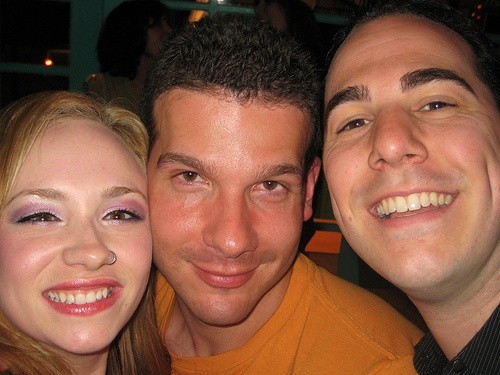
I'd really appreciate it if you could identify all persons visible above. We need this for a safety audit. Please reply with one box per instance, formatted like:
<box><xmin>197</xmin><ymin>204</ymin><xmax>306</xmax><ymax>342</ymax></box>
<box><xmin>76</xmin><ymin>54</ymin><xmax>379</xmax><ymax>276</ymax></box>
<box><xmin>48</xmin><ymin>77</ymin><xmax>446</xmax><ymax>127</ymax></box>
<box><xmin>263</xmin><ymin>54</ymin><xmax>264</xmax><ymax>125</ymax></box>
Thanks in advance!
<box><xmin>315</xmin><ymin>0</ymin><xmax>500</xmax><ymax>374</ymax></box>
<box><xmin>0</xmin><ymin>88</ymin><xmax>173</xmax><ymax>375</ymax></box>
<box><xmin>141</xmin><ymin>10</ymin><xmax>428</xmax><ymax>375</ymax></box>
